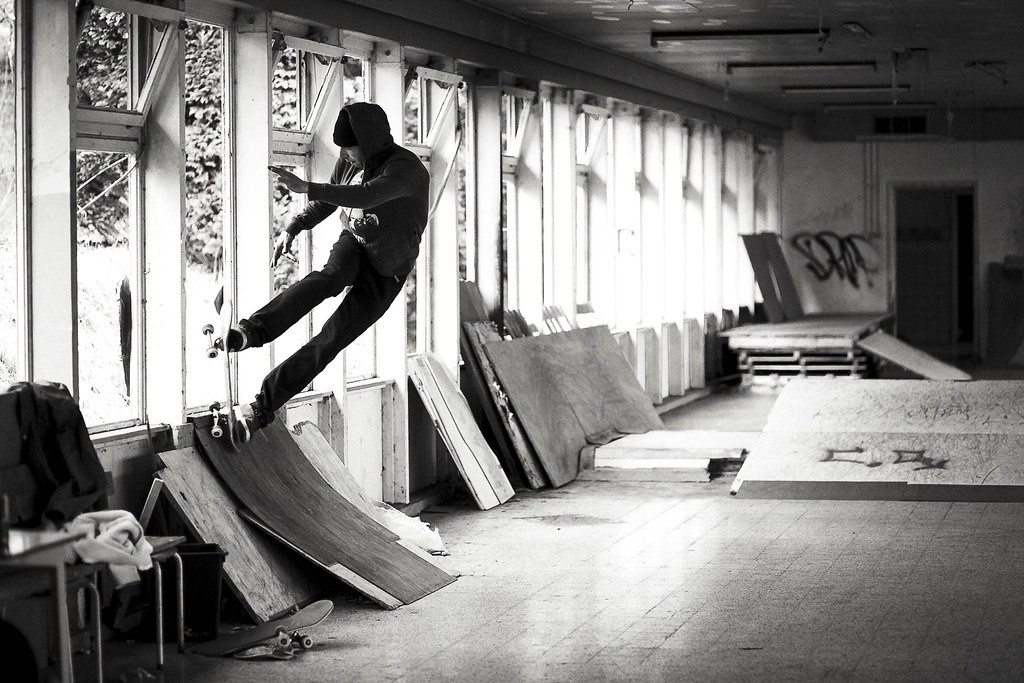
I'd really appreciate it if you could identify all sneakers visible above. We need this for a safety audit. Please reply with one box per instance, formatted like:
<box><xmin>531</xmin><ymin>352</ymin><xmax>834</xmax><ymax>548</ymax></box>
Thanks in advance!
<box><xmin>232</xmin><ymin>404</ymin><xmax>260</xmax><ymax>443</ymax></box>
<box><xmin>228</xmin><ymin>326</ymin><xmax>251</xmax><ymax>352</ymax></box>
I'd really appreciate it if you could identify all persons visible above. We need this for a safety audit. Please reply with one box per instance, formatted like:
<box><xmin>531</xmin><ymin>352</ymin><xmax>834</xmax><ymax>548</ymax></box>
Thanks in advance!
<box><xmin>229</xmin><ymin>102</ymin><xmax>431</xmax><ymax>444</ymax></box>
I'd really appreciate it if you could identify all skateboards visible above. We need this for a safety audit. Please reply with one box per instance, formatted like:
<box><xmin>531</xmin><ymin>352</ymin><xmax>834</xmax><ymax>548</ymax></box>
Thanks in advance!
<box><xmin>201</xmin><ymin>297</ymin><xmax>245</xmax><ymax>454</ymax></box>
<box><xmin>188</xmin><ymin>599</ymin><xmax>335</xmax><ymax>657</ymax></box>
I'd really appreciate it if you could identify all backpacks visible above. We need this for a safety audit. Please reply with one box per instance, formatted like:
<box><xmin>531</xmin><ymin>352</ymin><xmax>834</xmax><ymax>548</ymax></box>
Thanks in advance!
<box><xmin>0</xmin><ymin>379</ymin><xmax>109</xmax><ymax>530</ymax></box>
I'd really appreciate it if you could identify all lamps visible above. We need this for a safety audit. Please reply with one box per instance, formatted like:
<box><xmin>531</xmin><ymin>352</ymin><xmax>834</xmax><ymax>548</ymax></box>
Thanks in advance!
<box><xmin>649</xmin><ymin>29</ymin><xmax>834</xmax><ymax>47</ymax></box>
<box><xmin>824</xmin><ymin>103</ymin><xmax>936</xmax><ymax>112</ymax></box>
<box><xmin>781</xmin><ymin>87</ymin><xmax>909</xmax><ymax>97</ymax></box>
<box><xmin>726</xmin><ymin>62</ymin><xmax>879</xmax><ymax>74</ymax></box>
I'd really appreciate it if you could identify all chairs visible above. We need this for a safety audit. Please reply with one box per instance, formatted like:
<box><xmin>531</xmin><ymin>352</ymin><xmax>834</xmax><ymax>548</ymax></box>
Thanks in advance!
<box><xmin>0</xmin><ymin>379</ymin><xmax>185</xmax><ymax>683</ymax></box>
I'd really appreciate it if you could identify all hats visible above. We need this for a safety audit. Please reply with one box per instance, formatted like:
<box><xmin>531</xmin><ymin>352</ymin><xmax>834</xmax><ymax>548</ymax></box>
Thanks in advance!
<box><xmin>333</xmin><ymin>109</ymin><xmax>359</xmax><ymax>147</ymax></box>
